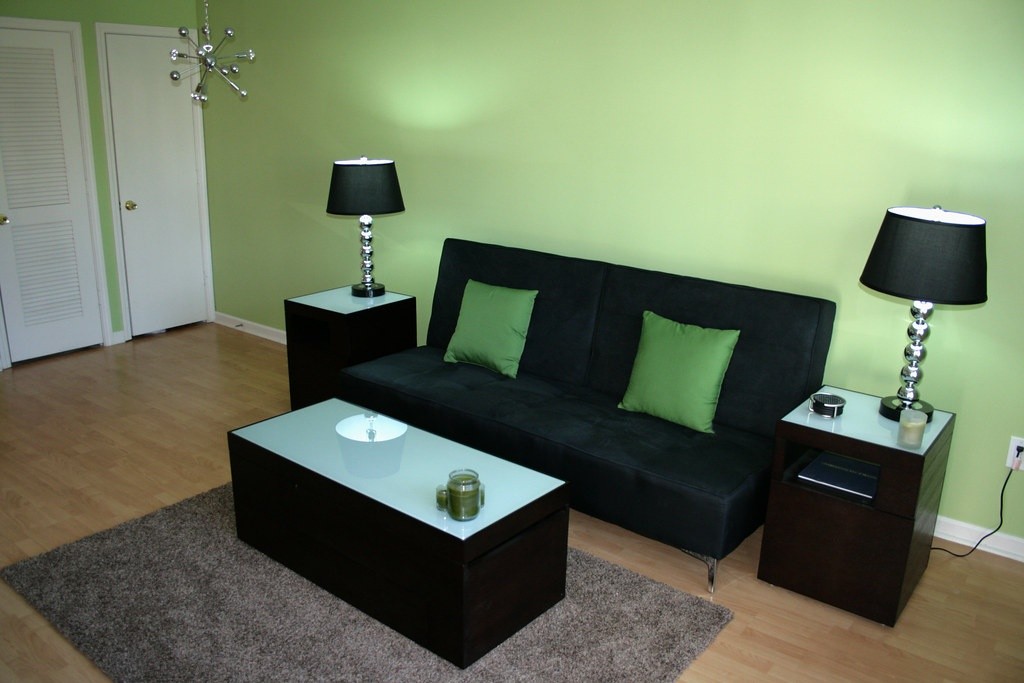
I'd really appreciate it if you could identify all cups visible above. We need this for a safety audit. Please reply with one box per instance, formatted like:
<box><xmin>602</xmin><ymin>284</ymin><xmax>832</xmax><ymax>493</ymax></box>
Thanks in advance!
<box><xmin>898</xmin><ymin>409</ymin><xmax>927</xmax><ymax>449</ymax></box>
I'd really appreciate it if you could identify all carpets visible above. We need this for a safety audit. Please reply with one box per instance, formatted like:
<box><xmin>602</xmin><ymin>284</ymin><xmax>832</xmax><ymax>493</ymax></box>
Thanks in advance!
<box><xmin>0</xmin><ymin>481</ymin><xmax>735</xmax><ymax>683</ymax></box>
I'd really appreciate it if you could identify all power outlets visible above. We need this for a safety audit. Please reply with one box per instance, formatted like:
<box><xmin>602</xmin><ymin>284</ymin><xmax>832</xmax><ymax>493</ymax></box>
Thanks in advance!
<box><xmin>1006</xmin><ymin>435</ymin><xmax>1024</xmax><ymax>471</ymax></box>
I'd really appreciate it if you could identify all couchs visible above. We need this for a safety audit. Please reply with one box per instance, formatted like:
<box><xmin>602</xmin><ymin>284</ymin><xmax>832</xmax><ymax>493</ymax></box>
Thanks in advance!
<box><xmin>333</xmin><ymin>238</ymin><xmax>837</xmax><ymax>595</ymax></box>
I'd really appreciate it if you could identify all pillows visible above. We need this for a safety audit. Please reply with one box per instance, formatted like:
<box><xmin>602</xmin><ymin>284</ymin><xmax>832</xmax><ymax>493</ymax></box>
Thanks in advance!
<box><xmin>618</xmin><ymin>311</ymin><xmax>741</xmax><ymax>433</ymax></box>
<box><xmin>442</xmin><ymin>278</ymin><xmax>539</xmax><ymax>381</ymax></box>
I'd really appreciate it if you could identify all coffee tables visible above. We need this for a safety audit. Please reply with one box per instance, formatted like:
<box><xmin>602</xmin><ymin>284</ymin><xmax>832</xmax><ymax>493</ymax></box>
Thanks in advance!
<box><xmin>226</xmin><ymin>398</ymin><xmax>573</xmax><ymax>673</ymax></box>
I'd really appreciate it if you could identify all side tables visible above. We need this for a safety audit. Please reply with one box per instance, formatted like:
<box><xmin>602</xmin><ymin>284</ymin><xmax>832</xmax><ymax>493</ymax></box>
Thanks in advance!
<box><xmin>283</xmin><ymin>285</ymin><xmax>418</xmax><ymax>411</ymax></box>
<box><xmin>756</xmin><ymin>385</ymin><xmax>957</xmax><ymax>629</ymax></box>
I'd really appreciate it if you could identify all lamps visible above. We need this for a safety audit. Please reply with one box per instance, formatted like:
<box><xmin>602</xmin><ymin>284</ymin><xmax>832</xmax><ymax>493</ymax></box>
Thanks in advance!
<box><xmin>169</xmin><ymin>0</ymin><xmax>257</xmax><ymax>102</ymax></box>
<box><xmin>335</xmin><ymin>410</ymin><xmax>408</xmax><ymax>480</ymax></box>
<box><xmin>325</xmin><ymin>156</ymin><xmax>406</xmax><ymax>298</ymax></box>
<box><xmin>859</xmin><ymin>204</ymin><xmax>989</xmax><ymax>423</ymax></box>
